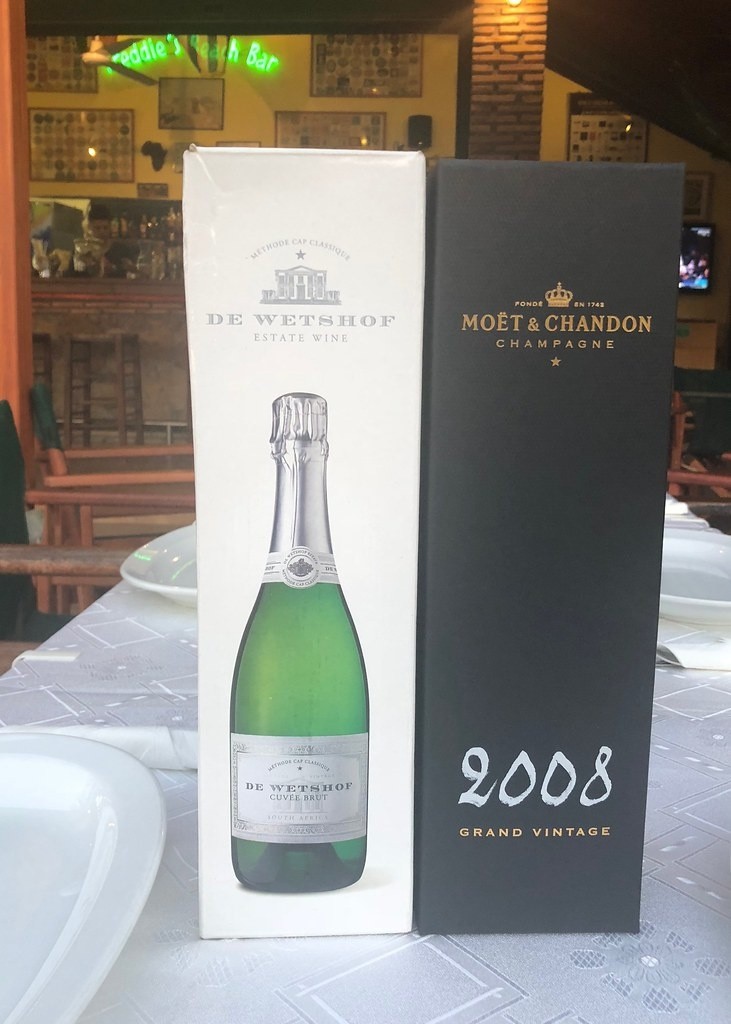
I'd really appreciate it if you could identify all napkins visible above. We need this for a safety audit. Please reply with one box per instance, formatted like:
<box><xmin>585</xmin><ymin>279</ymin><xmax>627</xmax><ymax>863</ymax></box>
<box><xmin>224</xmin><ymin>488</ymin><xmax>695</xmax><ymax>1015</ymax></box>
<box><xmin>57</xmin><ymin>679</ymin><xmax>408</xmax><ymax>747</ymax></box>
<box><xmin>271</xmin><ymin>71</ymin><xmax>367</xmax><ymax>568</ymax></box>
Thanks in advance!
<box><xmin>0</xmin><ymin>725</ymin><xmax>199</xmax><ymax>771</ymax></box>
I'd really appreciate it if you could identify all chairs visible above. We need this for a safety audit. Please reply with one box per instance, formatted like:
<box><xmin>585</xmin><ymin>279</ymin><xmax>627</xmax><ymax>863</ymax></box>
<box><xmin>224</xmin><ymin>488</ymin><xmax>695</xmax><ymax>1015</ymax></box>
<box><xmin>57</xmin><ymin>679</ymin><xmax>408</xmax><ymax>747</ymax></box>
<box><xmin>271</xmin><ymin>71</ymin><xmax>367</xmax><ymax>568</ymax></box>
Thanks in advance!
<box><xmin>666</xmin><ymin>388</ymin><xmax>731</xmax><ymax>534</ymax></box>
<box><xmin>0</xmin><ymin>383</ymin><xmax>197</xmax><ymax>676</ymax></box>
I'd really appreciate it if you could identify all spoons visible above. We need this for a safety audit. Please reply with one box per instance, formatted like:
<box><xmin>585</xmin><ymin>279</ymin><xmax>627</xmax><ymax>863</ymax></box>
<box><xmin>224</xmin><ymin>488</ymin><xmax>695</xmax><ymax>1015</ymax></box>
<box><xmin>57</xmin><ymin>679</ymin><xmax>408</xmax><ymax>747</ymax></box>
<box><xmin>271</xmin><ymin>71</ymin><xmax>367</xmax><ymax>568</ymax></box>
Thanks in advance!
<box><xmin>656</xmin><ymin>643</ymin><xmax>679</xmax><ymax>665</ymax></box>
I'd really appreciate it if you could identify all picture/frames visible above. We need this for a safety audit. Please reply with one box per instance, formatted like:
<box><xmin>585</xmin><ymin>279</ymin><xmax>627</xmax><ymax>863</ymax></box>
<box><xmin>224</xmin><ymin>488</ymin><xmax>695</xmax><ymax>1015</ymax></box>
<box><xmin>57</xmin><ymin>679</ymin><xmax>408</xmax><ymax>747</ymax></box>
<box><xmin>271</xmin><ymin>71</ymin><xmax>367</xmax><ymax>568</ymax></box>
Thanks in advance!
<box><xmin>681</xmin><ymin>171</ymin><xmax>713</xmax><ymax>224</ymax></box>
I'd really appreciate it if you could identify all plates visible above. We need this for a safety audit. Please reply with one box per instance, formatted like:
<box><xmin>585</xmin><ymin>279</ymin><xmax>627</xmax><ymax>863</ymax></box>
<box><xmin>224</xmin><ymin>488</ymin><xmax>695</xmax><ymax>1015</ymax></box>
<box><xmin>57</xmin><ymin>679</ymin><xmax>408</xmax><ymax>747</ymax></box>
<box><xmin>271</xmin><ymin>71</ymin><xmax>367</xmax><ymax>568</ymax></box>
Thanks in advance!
<box><xmin>120</xmin><ymin>523</ymin><xmax>196</xmax><ymax>606</ymax></box>
<box><xmin>0</xmin><ymin>731</ymin><xmax>167</xmax><ymax>1024</ymax></box>
<box><xmin>657</xmin><ymin>528</ymin><xmax>731</xmax><ymax>630</ymax></box>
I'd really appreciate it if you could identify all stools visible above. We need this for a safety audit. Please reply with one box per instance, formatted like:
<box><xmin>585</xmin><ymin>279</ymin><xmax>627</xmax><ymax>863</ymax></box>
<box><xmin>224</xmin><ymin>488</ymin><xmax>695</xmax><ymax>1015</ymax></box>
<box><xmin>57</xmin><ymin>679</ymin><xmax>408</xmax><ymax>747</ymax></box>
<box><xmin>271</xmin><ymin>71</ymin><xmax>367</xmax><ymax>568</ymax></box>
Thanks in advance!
<box><xmin>64</xmin><ymin>333</ymin><xmax>144</xmax><ymax>451</ymax></box>
<box><xmin>33</xmin><ymin>333</ymin><xmax>53</xmax><ymax>405</ymax></box>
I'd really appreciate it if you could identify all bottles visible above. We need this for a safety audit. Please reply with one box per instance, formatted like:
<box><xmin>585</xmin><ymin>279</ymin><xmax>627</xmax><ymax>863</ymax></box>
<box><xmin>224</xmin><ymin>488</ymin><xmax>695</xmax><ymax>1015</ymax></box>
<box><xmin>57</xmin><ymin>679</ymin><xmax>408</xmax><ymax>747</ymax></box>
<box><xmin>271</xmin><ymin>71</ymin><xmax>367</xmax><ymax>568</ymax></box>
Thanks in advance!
<box><xmin>228</xmin><ymin>392</ymin><xmax>371</xmax><ymax>891</ymax></box>
<box><xmin>137</xmin><ymin>239</ymin><xmax>167</xmax><ymax>282</ymax></box>
<box><xmin>72</xmin><ymin>237</ymin><xmax>108</xmax><ymax>282</ymax></box>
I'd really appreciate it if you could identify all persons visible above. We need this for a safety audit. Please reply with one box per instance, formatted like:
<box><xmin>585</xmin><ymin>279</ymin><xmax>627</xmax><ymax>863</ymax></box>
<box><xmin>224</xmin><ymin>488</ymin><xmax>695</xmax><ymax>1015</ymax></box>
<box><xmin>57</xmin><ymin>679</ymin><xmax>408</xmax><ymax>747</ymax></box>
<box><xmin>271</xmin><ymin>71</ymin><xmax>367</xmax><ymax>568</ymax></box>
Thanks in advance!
<box><xmin>68</xmin><ymin>204</ymin><xmax>141</xmax><ymax>375</ymax></box>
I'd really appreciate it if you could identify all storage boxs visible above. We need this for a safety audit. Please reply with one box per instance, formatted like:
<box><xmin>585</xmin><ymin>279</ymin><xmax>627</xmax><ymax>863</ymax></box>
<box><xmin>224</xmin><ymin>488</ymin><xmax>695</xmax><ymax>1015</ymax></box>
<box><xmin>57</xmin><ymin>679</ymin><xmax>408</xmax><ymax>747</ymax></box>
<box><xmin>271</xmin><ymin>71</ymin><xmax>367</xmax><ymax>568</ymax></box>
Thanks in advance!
<box><xmin>181</xmin><ymin>145</ymin><xmax>424</xmax><ymax>937</ymax></box>
<box><xmin>410</xmin><ymin>158</ymin><xmax>686</xmax><ymax>937</ymax></box>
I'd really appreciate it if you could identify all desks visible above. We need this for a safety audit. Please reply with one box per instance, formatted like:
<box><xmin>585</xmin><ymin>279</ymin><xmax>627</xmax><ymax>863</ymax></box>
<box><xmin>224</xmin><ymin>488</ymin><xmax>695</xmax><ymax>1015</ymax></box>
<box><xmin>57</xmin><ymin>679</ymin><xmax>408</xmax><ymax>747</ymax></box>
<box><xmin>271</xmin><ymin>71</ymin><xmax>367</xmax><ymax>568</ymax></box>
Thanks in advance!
<box><xmin>0</xmin><ymin>490</ymin><xmax>731</xmax><ymax>1024</ymax></box>
